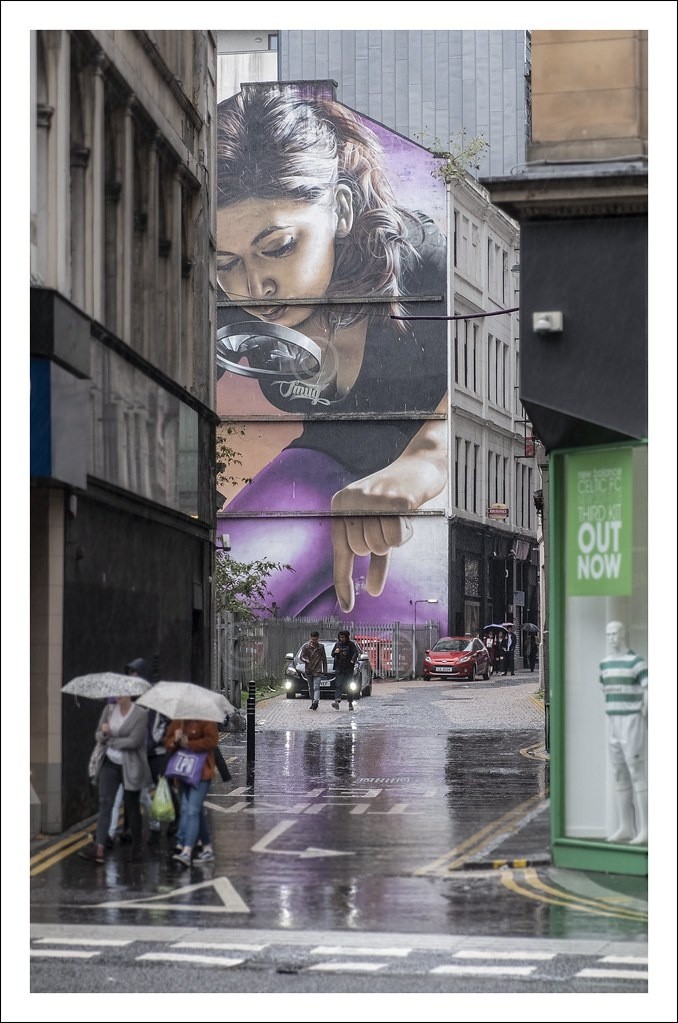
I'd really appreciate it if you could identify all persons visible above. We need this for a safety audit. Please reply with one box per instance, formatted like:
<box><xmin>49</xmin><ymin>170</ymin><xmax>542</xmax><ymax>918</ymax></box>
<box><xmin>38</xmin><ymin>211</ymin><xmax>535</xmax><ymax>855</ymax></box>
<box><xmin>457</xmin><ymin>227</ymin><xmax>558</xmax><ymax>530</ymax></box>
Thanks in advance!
<box><xmin>300</xmin><ymin>631</ymin><xmax>327</xmax><ymax>711</ymax></box>
<box><xmin>77</xmin><ymin>697</ymin><xmax>153</xmax><ymax>865</ymax></box>
<box><xmin>598</xmin><ymin>621</ymin><xmax>648</xmax><ymax>845</ymax></box>
<box><xmin>331</xmin><ymin>630</ymin><xmax>359</xmax><ymax>710</ymax></box>
<box><xmin>528</xmin><ymin>635</ymin><xmax>538</xmax><ymax>672</ymax></box>
<box><xmin>483</xmin><ymin>626</ymin><xmax>517</xmax><ymax>676</ymax></box>
<box><xmin>88</xmin><ymin>658</ymin><xmax>233</xmax><ymax>851</ymax></box>
<box><xmin>161</xmin><ymin>720</ymin><xmax>219</xmax><ymax>868</ymax></box>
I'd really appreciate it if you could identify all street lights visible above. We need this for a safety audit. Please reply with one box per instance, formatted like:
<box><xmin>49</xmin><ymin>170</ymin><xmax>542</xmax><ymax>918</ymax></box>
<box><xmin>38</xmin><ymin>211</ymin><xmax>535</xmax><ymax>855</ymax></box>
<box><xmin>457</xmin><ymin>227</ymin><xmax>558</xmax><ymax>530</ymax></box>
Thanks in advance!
<box><xmin>412</xmin><ymin>599</ymin><xmax>438</xmax><ymax>680</ymax></box>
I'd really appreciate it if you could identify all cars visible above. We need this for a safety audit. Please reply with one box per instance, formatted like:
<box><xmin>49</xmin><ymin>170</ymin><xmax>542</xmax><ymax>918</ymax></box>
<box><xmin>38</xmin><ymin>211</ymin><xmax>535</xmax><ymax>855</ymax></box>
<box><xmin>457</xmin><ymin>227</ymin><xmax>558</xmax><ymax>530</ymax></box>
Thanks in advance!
<box><xmin>422</xmin><ymin>636</ymin><xmax>492</xmax><ymax>682</ymax></box>
<box><xmin>284</xmin><ymin>639</ymin><xmax>373</xmax><ymax>700</ymax></box>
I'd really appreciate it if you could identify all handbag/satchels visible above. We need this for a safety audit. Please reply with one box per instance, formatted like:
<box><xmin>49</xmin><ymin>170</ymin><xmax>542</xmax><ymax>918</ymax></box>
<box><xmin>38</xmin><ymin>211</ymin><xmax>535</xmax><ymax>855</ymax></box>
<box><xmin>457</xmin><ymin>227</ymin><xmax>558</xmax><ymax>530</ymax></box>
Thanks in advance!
<box><xmin>88</xmin><ymin>742</ymin><xmax>103</xmax><ymax>778</ymax></box>
<box><xmin>164</xmin><ymin>749</ymin><xmax>209</xmax><ymax>784</ymax></box>
<box><xmin>148</xmin><ymin>775</ymin><xmax>175</xmax><ymax>822</ymax></box>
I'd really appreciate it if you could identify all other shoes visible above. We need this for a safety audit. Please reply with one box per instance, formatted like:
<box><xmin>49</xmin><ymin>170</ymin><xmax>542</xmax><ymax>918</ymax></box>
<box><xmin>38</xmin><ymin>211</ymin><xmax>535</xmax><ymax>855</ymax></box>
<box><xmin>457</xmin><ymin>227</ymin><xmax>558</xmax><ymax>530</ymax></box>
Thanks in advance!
<box><xmin>511</xmin><ymin>672</ymin><xmax>515</xmax><ymax>675</ymax></box>
<box><xmin>310</xmin><ymin>702</ymin><xmax>319</xmax><ymax>710</ymax></box>
<box><xmin>331</xmin><ymin>701</ymin><xmax>339</xmax><ymax>710</ymax></box>
<box><xmin>192</xmin><ymin>850</ymin><xmax>216</xmax><ymax>863</ymax></box>
<box><xmin>145</xmin><ymin>825</ymin><xmax>161</xmax><ymax>834</ymax></box>
<box><xmin>85</xmin><ymin>830</ymin><xmax>113</xmax><ymax>849</ymax></box>
<box><xmin>77</xmin><ymin>844</ymin><xmax>104</xmax><ymax>864</ymax></box>
<box><xmin>502</xmin><ymin>672</ymin><xmax>507</xmax><ymax>676</ymax></box>
<box><xmin>172</xmin><ymin>852</ymin><xmax>191</xmax><ymax>867</ymax></box>
<box><xmin>349</xmin><ymin>703</ymin><xmax>354</xmax><ymax>710</ymax></box>
<box><xmin>120</xmin><ymin>831</ymin><xmax>134</xmax><ymax>842</ymax></box>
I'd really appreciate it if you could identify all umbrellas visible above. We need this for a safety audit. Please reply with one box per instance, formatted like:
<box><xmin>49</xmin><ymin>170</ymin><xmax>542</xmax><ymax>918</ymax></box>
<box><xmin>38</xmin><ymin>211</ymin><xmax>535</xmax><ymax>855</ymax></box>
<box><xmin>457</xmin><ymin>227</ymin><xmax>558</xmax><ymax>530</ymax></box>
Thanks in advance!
<box><xmin>60</xmin><ymin>672</ymin><xmax>152</xmax><ymax>724</ymax></box>
<box><xmin>484</xmin><ymin>624</ymin><xmax>508</xmax><ymax>633</ymax></box>
<box><xmin>134</xmin><ymin>680</ymin><xmax>237</xmax><ymax>730</ymax></box>
<box><xmin>501</xmin><ymin>623</ymin><xmax>514</xmax><ymax>626</ymax></box>
<box><xmin>517</xmin><ymin>623</ymin><xmax>540</xmax><ymax>631</ymax></box>
